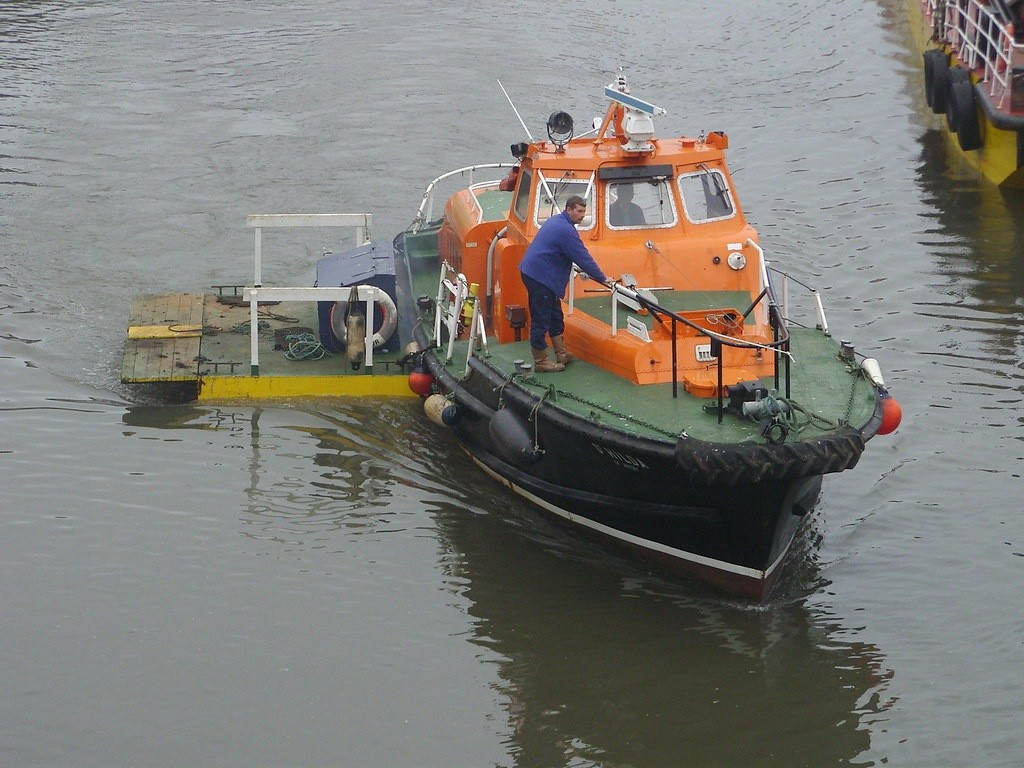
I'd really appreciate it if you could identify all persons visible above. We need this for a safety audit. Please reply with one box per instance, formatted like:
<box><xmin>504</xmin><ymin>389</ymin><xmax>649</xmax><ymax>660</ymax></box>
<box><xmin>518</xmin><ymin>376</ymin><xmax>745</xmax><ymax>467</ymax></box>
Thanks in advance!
<box><xmin>609</xmin><ymin>183</ymin><xmax>645</xmax><ymax>226</ymax></box>
<box><xmin>518</xmin><ymin>195</ymin><xmax>614</xmax><ymax>371</ymax></box>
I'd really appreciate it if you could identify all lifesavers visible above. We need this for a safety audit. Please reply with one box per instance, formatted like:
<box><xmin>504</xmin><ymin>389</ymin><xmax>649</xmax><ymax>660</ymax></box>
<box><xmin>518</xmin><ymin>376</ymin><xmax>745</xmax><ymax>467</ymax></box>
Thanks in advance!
<box><xmin>943</xmin><ymin>68</ymin><xmax>983</xmax><ymax>151</ymax></box>
<box><xmin>923</xmin><ymin>48</ymin><xmax>948</xmax><ymax>114</ymax></box>
<box><xmin>331</xmin><ymin>285</ymin><xmax>397</xmax><ymax>349</ymax></box>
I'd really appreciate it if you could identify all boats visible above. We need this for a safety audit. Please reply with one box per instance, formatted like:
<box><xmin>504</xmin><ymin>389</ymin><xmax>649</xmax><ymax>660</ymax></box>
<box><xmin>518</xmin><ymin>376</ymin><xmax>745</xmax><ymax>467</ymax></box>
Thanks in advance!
<box><xmin>903</xmin><ymin>0</ymin><xmax>1024</xmax><ymax>194</ymax></box>
<box><xmin>389</xmin><ymin>64</ymin><xmax>886</xmax><ymax>607</ymax></box>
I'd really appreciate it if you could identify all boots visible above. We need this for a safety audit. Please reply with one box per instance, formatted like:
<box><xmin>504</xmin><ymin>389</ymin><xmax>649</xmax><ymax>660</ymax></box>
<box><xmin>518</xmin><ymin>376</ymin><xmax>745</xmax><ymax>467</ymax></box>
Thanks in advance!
<box><xmin>551</xmin><ymin>333</ymin><xmax>573</xmax><ymax>364</ymax></box>
<box><xmin>531</xmin><ymin>346</ymin><xmax>565</xmax><ymax>372</ymax></box>
